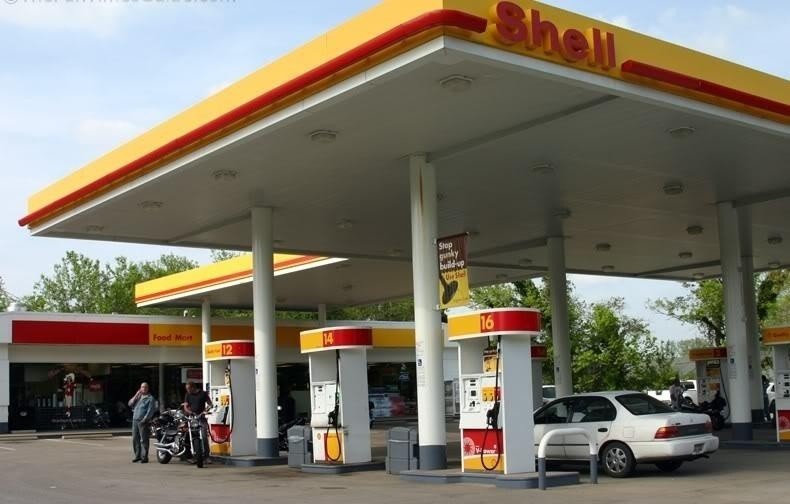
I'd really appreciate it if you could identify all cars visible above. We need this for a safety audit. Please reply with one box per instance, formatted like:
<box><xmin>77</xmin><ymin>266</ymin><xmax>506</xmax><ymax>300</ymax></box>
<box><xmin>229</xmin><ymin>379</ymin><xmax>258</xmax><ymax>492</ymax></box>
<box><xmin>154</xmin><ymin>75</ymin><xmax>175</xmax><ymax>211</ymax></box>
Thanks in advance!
<box><xmin>533</xmin><ymin>390</ymin><xmax>720</xmax><ymax>478</ymax></box>
<box><xmin>766</xmin><ymin>382</ymin><xmax>775</xmax><ymax>413</ymax></box>
<box><xmin>643</xmin><ymin>380</ymin><xmax>698</xmax><ymax>406</ymax></box>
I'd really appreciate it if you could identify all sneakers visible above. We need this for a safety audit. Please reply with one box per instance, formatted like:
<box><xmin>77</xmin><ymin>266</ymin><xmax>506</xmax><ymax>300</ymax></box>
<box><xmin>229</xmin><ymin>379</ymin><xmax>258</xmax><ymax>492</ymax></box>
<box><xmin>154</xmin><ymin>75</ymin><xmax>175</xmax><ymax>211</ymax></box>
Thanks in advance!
<box><xmin>205</xmin><ymin>458</ymin><xmax>213</xmax><ymax>465</ymax></box>
<box><xmin>132</xmin><ymin>456</ymin><xmax>149</xmax><ymax>463</ymax></box>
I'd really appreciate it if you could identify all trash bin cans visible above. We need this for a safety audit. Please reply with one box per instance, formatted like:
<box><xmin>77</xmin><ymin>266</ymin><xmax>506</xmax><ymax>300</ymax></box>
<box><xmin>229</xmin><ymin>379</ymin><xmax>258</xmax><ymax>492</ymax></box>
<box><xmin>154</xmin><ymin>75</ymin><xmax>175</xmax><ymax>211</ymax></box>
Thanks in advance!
<box><xmin>287</xmin><ymin>425</ymin><xmax>312</xmax><ymax>468</ymax></box>
<box><xmin>385</xmin><ymin>427</ymin><xmax>418</xmax><ymax>474</ymax></box>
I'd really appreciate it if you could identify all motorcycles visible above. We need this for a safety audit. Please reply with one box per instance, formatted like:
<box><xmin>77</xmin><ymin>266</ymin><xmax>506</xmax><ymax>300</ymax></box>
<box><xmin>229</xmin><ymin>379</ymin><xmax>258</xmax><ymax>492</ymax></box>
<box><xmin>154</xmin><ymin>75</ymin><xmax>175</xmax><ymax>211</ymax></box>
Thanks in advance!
<box><xmin>679</xmin><ymin>390</ymin><xmax>726</xmax><ymax>430</ymax></box>
<box><xmin>279</xmin><ymin>416</ymin><xmax>306</xmax><ymax>452</ymax></box>
<box><xmin>150</xmin><ymin>405</ymin><xmax>212</xmax><ymax>468</ymax></box>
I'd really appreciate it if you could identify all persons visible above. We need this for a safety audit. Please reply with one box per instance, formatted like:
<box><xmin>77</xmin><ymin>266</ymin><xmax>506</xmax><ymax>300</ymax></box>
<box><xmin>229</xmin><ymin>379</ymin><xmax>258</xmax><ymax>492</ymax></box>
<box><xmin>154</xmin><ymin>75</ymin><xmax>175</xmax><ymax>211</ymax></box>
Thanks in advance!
<box><xmin>183</xmin><ymin>379</ymin><xmax>213</xmax><ymax>464</ymax></box>
<box><xmin>128</xmin><ymin>381</ymin><xmax>156</xmax><ymax>463</ymax></box>
<box><xmin>668</xmin><ymin>376</ymin><xmax>683</xmax><ymax>410</ymax></box>
<box><xmin>276</xmin><ymin>383</ymin><xmax>297</xmax><ymax>451</ymax></box>
<box><xmin>762</xmin><ymin>373</ymin><xmax>772</xmax><ymax>421</ymax></box>
<box><xmin>62</xmin><ymin>375</ymin><xmax>77</xmax><ymax>417</ymax></box>
